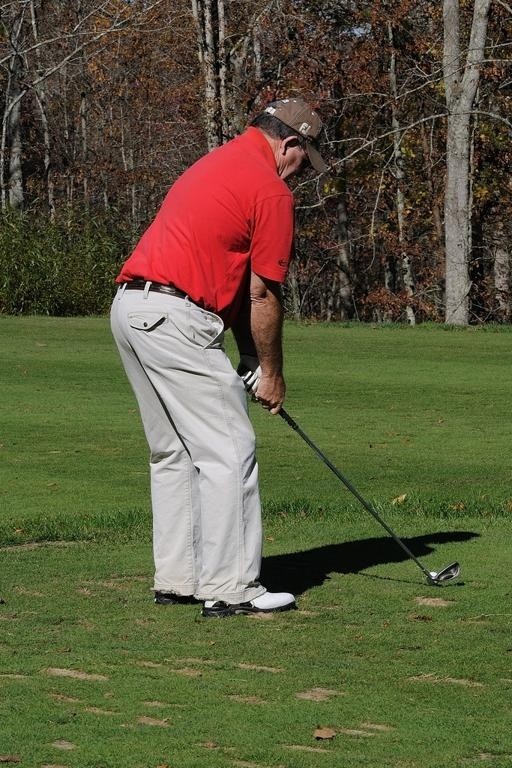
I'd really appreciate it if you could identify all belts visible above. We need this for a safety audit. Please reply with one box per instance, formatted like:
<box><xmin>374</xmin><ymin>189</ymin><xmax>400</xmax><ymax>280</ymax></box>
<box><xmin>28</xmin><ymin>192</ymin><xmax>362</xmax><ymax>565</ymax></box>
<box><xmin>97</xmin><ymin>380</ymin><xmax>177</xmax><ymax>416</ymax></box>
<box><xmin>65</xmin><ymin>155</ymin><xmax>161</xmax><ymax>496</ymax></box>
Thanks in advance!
<box><xmin>119</xmin><ymin>279</ymin><xmax>197</xmax><ymax>304</ymax></box>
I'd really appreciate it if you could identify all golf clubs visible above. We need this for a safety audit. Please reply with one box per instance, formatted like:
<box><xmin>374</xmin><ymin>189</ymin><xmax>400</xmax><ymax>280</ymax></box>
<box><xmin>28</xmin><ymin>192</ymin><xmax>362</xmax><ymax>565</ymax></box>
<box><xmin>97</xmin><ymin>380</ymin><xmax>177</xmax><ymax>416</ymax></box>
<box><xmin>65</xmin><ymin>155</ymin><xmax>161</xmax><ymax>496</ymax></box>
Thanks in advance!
<box><xmin>237</xmin><ymin>362</ymin><xmax>461</xmax><ymax>589</ymax></box>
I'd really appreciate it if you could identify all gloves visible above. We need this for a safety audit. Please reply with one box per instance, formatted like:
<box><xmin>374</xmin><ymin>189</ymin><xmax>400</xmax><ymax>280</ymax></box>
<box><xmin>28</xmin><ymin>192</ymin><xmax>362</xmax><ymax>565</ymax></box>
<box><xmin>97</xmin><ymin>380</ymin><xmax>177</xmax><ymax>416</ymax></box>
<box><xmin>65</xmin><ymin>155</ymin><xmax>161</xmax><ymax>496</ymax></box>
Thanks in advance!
<box><xmin>236</xmin><ymin>351</ymin><xmax>262</xmax><ymax>403</ymax></box>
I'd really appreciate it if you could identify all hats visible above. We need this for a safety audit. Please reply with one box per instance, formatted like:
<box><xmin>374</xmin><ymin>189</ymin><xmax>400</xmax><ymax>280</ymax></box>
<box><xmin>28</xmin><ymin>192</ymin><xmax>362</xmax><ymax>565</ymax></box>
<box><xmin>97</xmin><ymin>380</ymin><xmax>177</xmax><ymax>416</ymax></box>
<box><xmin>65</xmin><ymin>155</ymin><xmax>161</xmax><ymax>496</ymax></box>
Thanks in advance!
<box><xmin>264</xmin><ymin>95</ymin><xmax>329</xmax><ymax>174</ymax></box>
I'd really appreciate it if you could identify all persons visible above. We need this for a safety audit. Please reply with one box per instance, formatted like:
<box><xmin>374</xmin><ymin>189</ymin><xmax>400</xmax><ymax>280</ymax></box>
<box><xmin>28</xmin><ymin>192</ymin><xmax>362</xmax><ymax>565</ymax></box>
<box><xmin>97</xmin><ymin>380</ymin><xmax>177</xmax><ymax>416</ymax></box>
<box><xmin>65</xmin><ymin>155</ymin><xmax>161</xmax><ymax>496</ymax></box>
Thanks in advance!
<box><xmin>109</xmin><ymin>95</ymin><xmax>329</xmax><ymax>617</ymax></box>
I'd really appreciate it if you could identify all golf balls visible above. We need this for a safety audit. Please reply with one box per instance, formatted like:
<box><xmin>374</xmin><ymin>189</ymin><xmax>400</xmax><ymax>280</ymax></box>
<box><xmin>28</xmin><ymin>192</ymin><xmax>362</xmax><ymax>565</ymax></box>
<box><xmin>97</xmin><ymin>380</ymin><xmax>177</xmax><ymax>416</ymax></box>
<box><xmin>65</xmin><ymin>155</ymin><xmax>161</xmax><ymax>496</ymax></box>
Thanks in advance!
<box><xmin>429</xmin><ymin>571</ymin><xmax>438</xmax><ymax>580</ymax></box>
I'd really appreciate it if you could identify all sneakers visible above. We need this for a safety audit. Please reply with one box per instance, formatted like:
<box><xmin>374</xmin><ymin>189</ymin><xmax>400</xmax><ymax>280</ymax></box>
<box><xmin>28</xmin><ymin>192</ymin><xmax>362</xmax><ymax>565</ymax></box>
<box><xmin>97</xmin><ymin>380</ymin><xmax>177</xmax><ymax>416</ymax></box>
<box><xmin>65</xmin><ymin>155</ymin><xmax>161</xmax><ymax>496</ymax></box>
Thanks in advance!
<box><xmin>155</xmin><ymin>590</ymin><xmax>204</xmax><ymax>606</ymax></box>
<box><xmin>201</xmin><ymin>589</ymin><xmax>297</xmax><ymax>618</ymax></box>
